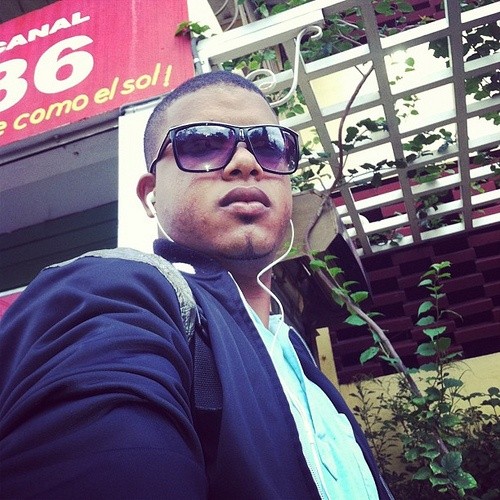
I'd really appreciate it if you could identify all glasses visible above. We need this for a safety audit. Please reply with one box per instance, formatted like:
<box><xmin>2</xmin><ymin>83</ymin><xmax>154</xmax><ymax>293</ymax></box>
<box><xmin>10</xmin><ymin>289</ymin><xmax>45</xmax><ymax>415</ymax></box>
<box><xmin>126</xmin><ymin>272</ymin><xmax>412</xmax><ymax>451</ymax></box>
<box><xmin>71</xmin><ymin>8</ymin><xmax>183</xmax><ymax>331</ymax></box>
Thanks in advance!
<box><xmin>149</xmin><ymin>120</ymin><xmax>299</xmax><ymax>176</ymax></box>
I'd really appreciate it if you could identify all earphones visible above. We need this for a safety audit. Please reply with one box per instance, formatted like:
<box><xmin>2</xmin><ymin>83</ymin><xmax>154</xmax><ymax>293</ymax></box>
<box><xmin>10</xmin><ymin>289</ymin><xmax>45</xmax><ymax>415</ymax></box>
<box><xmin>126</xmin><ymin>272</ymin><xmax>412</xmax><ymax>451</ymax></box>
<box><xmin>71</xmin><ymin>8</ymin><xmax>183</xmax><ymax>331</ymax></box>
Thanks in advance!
<box><xmin>145</xmin><ymin>191</ymin><xmax>156</xmax><ymax>215</ymax></box>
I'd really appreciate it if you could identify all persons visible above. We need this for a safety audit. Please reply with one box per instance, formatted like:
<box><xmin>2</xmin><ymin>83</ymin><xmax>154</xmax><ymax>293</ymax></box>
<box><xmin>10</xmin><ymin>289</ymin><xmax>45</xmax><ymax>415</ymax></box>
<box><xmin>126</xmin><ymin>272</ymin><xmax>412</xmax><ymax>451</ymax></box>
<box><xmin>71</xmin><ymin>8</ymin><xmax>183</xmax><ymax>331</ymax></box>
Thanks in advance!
<box><xmin>1</xmin><ymin>71</ymin><xmax>393</xmax><ymax>500</ymax></box>
